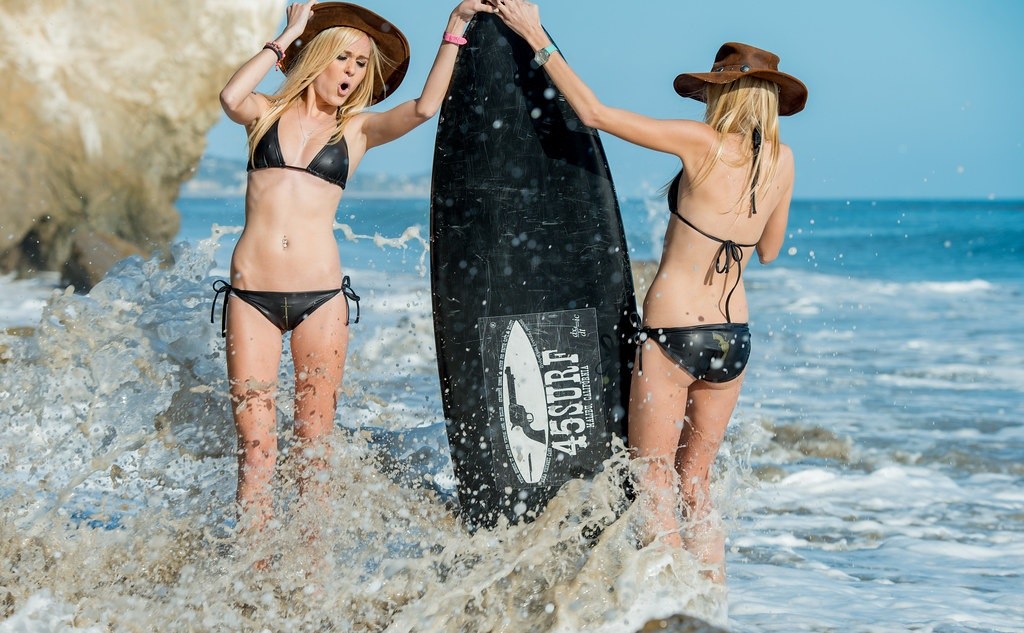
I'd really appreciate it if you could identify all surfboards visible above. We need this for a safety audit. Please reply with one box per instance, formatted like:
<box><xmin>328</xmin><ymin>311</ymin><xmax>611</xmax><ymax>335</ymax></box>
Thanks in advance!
<box><xmin>428</xmin><ymin>1</ymin><xmax>638</xmax><ymax>525</ymax></box>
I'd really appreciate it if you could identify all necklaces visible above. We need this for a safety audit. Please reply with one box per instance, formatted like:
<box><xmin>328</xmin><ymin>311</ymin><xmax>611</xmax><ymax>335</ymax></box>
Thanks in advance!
<box><xmin>297</xmin><ymin>98</ymin><xmax>324</xmax><ymax>145</ymax></box>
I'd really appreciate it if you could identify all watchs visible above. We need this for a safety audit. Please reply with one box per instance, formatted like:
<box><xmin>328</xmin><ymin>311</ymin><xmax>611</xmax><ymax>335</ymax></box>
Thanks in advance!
<box><xmin>530</xmin><ymin>44</ymin><xmax>559</xmax><ymax>70</ymax></box>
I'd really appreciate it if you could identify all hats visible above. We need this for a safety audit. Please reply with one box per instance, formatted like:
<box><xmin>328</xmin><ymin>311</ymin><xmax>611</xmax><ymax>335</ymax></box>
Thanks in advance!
<box><xmin>673</xmin><ymin>42</ymin><xmax>808</xmax><ymax>117</ymax></box>
<box><xmin>278</xmin><ymin>2</ymin><xmax>410</xmax><ymax>106</ymax></box>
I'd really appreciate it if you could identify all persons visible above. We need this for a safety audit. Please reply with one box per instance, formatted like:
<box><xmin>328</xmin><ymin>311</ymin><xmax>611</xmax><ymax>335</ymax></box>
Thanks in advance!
<box><xmin>210</xmin><ymin>0</ymin><xmax>500</xmax><ymax>570</ymax></box>
<box><xmin>488</xmin><ymin>0</ymin><xmax>809</xmax><ymax>587</ymax></box>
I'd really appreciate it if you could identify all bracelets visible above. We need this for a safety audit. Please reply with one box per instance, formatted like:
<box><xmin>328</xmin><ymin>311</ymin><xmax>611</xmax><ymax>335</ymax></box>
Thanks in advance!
<box><xmin>263</xmin><ymin>41</ymin><xmax>286</xmax><ymax>71</ymax></box>
<box><xmin>442</xmin><ymin>32</ymin><xmax>467</xmax><ymax>45</ymax></box>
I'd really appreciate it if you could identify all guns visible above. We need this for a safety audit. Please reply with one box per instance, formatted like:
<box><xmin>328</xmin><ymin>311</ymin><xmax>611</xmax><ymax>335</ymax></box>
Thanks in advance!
<box><xmin>503</xmin><ymin>367</ymin><xmax>548</xmax><ymax>446</ymax></box>
